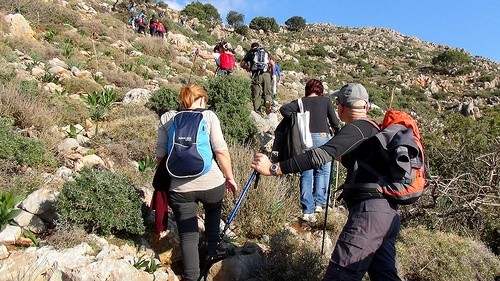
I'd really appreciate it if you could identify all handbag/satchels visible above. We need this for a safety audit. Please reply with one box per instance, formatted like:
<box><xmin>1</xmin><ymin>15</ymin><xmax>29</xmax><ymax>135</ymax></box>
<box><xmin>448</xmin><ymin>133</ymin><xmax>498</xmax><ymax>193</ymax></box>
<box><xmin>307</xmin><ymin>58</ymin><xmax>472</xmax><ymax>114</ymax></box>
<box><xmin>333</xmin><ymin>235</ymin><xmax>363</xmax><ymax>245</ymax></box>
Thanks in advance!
<box><xmin>297</xmin><ymin>98</ymin><xmax>313</xmax><ymax>149</ymax></box>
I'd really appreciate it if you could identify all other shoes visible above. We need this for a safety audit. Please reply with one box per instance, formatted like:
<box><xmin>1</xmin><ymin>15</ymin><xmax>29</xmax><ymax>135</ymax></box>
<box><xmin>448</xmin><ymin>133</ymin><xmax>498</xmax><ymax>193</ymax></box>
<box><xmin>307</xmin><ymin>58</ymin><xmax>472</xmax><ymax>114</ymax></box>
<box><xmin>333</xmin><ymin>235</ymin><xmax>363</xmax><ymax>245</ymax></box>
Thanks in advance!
<box><xmin>303</xmin><ymin>212</ymin><xmax>316</xmax><ymax>223</ymax></box>
<box><xmin>265</xmin><ymin>101</ymin><xmax>271</xmax><ymax>114</ymax></box>
<box><xmin>205</xmin><ymin>250</ymin><xmax>227</xmax><ymax>262</ymax></box>
<box><xmin>315</xmin><ymin>205</ymin><xmax>333</xmax><ymax>213</ymax></box>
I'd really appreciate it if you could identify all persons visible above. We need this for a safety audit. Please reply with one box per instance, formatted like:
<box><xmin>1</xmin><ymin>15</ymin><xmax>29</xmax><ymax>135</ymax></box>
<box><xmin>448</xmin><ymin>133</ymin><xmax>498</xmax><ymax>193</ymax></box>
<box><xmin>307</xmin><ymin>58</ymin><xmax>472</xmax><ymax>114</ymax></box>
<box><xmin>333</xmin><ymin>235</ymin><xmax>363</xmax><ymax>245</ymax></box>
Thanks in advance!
<box><xmin>251</xmin><ymin>82</ymin><xmax>402</xmax><ymax>281</ymax></box>
<box><xmin>179</xmin><ymin>16</ymin><xmax>187</xmax><ymax>25</ymax></box>
<box><xmin>154</xmin><ymin>84</ymin><xmax>237</xmax><ymax>281</ymax></box>
<box><xmin>280</xmin><ymin>78</ymin><xmax>341</xmax><ymax>222</ymax></box>
<box><xmin>128</xmin><ymin>2</ymin><xmax>166</xmax><ymax>39</ymax></box>
<box><xmin>196</xmin><ymin>42</ymin><xmax>232</xmax><ymax>76</ymax></box>
<box><xmin>270</xmin><ymin>58</ymin><xmax>281</xmax><ymax>98</ymax></box>
<box><xmin>240</xmin><ymin>42</ymin><xmax>272</xmax><ymax>115</ymax></box>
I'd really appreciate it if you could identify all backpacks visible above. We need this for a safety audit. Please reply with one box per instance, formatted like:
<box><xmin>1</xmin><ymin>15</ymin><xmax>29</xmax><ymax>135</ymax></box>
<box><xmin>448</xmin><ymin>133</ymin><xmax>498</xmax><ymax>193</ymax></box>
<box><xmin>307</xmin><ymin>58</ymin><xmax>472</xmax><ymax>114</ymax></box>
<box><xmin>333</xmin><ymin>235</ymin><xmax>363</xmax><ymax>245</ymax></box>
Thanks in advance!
<box><xmin>220</xmin><ymin>52</ymin><xmax>234</xmax><ymax>69</ymax></box>
<box><xmin>249</xmin><ymin>47</ymin><xmax>270</xmax><ymax>71</ymax></box>
<box><xmin>165</xmin><ymin>107</ymin><xmax>213</xmax><ymax>179</ymax></box>
<box><xmin>356</xmin><ymin>110</ymin><xmax>424</xmax><ymax>205</ymax></box>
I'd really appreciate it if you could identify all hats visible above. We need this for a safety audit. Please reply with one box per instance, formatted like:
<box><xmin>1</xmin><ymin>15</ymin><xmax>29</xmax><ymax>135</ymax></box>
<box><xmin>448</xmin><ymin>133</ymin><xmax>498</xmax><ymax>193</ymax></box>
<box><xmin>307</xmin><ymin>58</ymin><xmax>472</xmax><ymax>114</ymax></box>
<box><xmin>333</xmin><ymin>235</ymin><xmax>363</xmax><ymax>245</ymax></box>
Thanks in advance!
<box><xmin>330</xmin><ymin>83</ymin><xmax>369</xmax><ymax>109</ymax></box>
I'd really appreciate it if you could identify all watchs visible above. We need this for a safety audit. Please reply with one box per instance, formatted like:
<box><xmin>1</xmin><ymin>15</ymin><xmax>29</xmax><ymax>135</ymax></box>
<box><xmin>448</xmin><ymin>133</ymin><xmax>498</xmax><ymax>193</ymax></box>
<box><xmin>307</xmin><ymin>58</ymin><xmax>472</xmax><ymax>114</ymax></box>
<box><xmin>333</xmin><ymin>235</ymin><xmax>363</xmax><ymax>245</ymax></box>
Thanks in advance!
<box><xmin>270</xmin><ymin>162</ymin><xmax>279</xmax><ymax>176</ymax></box>
